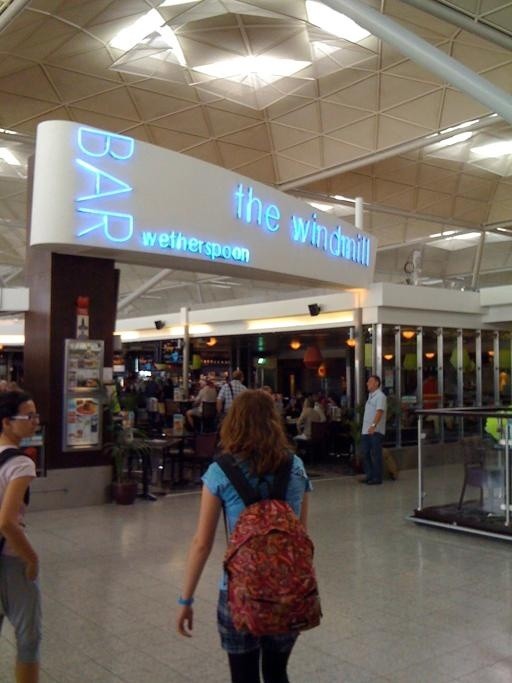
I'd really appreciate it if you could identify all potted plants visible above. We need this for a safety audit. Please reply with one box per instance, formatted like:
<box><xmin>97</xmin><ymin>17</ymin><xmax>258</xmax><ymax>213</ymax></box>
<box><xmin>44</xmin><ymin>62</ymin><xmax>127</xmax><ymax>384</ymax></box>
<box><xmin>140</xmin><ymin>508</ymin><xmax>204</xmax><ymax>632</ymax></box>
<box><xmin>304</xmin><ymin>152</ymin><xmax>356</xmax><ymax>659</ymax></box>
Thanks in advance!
<box><xmin>103</xmin><ymin>429</ymin><xmax>153</xmax><ymax>504</ymax></box>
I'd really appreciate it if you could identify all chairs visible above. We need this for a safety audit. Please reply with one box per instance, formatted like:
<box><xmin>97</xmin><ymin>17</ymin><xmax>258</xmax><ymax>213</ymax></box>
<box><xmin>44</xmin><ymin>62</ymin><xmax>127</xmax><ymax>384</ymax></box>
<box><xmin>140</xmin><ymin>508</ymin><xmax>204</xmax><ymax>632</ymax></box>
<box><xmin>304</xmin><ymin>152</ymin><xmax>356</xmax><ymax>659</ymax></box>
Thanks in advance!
<box><xmin>458</xmin><ymin>435</ymin><xmax>503</xmax><ymax>510</ymax></box>
<box><xmin>167</xmin><ymin>432</ymin><xmax>217</xmax><ymax>491</ymax></box>
<box><xmin>193</xmin><ymin>401</ymin><xmax>218</xmax><ymax>435</ymax></box>
<box><xmin>296</xmin><ymin>420</ymin><xmax>352</xmax><ymax>466</ymax></box>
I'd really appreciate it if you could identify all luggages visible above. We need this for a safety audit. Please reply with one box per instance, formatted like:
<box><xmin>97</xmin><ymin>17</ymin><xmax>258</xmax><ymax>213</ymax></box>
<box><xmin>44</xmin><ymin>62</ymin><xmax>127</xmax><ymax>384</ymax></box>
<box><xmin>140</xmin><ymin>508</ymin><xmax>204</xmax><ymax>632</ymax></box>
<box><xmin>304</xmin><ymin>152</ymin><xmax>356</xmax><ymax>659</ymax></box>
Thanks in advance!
<box><xmin>381</xmin><ymin>446</ymin><xmax>398</xmax><ymax>480</ymax></box>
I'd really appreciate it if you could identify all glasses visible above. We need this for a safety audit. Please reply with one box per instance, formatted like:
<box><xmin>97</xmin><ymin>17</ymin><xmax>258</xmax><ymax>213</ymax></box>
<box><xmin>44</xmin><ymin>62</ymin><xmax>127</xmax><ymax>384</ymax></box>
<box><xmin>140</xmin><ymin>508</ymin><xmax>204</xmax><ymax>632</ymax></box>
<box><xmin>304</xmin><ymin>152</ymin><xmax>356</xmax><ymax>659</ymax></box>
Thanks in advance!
<box><xmin>10</xmin><ymin>413</ymin><xmax>40</xmax><ymax>421</ymax></box>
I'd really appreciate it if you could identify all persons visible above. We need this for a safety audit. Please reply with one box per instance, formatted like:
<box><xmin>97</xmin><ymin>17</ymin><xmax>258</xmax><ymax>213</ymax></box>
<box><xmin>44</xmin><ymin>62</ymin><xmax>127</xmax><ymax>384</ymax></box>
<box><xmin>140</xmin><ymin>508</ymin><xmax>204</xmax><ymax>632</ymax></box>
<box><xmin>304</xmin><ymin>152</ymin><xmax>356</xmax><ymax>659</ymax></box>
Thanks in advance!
<box><xmin>0</xmin><ymin>388</ymin><xmax>42</xmax><ymax>681</ymax></box>
<box><xmin>356</xmin><ymin>374</ymin><xmax>390</xmax><ymax>484</ymax></box>
<box><xmin>176</xmin><ymin>387</ymin><xmax>314</xmax><ymax>683</ymax></box>
<box><xmin>131</xmin><ymin>363</ymin><xmax>352</xmax><ymax>457</ymax></box>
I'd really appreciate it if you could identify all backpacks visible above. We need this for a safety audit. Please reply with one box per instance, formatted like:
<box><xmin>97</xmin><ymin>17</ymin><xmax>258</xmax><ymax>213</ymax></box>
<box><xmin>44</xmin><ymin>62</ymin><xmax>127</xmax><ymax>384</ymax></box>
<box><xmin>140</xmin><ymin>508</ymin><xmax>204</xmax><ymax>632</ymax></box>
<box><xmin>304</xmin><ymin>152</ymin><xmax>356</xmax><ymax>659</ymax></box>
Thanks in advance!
<box><xmin>216</xmin><ymin>454</ymin><xmax>323</xmax><ymax>638</ymax></box>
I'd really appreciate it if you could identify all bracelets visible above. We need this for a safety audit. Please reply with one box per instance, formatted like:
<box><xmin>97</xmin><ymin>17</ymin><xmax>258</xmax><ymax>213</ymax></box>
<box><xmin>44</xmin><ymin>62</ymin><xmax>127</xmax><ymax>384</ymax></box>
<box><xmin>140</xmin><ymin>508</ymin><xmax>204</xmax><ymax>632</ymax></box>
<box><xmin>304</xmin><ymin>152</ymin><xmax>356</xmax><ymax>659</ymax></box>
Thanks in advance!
<box><xmin>370</xmin><ymin>422</ymin><xmax>376</xmax><ymax>426</ymax></box>
<box><xmin>178</xmin><ymin>595</ymin><xmax>194</xmax><ymax>606</ymax></box>
